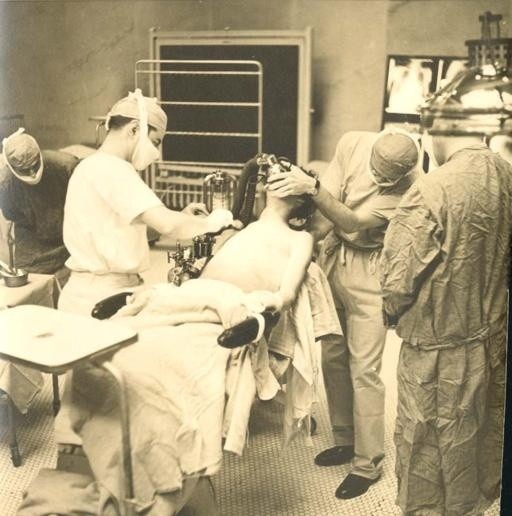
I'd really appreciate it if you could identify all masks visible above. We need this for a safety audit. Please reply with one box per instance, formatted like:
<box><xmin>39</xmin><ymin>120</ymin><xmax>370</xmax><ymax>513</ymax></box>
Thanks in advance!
<box><xmin>15</xmin><ymin>161</ymin><xmax>44</xmax><ymax>186</ymax></box>
<box><xmin>131</xmin><ymin>135</ymin><xmax>162</xmax><ymax>170</ymax></box>
<box><xmin>368</xmin><ymin>168</ymin><xmax>395</xmax><ymax>188</ymax></box>
<box><xmin>420</xmin><ymin>129</ymin><xmax>440</xmax><ymax>167</ymax></box>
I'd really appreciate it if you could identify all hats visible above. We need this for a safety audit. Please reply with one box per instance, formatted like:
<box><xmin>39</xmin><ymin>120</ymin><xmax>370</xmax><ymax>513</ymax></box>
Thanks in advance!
<box><xmin>4</xmin><ymin>135</ymin><xmax>40</xmax><ymax>170</ymax></box>
<box><xmin>109</xmin><ymin>94</ymin><xmax>168</xmax><ymax>139</ymax></box>
<box><xmin>369</xmin><ymin>132</ymin><xmax>418</xmax><ymax>181</ymax></box>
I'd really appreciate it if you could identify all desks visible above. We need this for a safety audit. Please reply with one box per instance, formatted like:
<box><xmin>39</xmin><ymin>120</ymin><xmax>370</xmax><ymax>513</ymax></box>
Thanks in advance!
<box><xmin>0</xmin><ymin>302</ymin><xmax>141</xmax><ymax>500</ymax></box>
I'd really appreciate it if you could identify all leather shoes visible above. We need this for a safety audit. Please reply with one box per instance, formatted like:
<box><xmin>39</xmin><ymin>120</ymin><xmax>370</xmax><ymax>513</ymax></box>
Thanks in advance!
<box><xmin>314</xmin><ymin>444</ymin><xmax>355</xmax><ymax>467</ymax></box>
<box><xmin>335</xmin><ymin>472</ymin><xmax>382</xmax><ymax>499</ymax></box>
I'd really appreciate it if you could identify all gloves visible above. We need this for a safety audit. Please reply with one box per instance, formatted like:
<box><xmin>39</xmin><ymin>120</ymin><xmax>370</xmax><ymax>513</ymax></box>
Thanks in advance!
<box><xmin>265</xmin><ymin>159</ymin><xmax>316</xmax><ymax>199</ymax></box>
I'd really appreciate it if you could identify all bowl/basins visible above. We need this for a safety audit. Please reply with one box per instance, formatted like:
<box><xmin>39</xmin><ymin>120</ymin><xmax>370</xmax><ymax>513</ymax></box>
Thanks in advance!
<box><xmin>3</xmin><ymin>269</ymin><xmax>27</xmax><ymax>287</ymax></box>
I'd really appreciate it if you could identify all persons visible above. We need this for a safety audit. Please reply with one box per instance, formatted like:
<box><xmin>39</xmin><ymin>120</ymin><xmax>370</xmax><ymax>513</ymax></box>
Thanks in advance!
<box><xmin>378</xmin><ymin>100</ymin><xmax>512</xmax><ymax>515</ymax></box>
<box><xmin>1</xmin><ymin>127</ymin><xmax>84</xmax><ymax>308</ymax></box>
<box><xmin>51</xmin><ymin>88</ymin><xmax>244</xmax><ymax>451</ymax></box>
<box><xmin>266</xmin><ymin>126</ymin><xmax>421</xmax><ymax>501</ymax></box>
<box><xmin>192</xmin><ymin>167</ymin><xmax>317</xmax><ymax>319</ymax></box>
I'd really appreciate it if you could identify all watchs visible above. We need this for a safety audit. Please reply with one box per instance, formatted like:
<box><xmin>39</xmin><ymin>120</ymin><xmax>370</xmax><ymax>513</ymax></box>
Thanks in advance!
<box><xmin>307</xmin><ymin>179</ymin><xmax>320</xmax><ymax>196</ymax></box>
